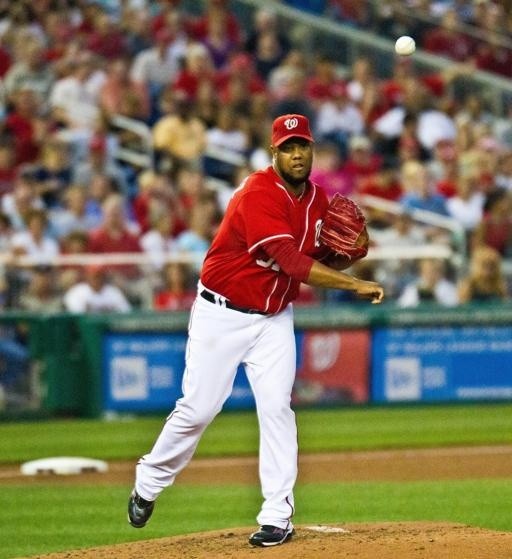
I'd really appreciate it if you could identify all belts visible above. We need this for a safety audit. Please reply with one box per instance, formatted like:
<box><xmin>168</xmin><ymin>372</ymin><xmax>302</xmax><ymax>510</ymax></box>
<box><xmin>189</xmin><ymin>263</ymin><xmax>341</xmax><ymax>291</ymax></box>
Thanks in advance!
<box><xmin>200</xmin><ymin>290</ymin><xmax>268</xmax><ymax>316</ymax></box>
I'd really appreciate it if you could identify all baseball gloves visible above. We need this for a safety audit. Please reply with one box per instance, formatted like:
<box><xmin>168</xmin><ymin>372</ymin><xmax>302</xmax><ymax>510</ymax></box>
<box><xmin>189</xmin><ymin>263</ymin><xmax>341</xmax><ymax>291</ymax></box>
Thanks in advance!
<box><xmin>318</xmin><ymin>191</ymin><xmax>371</xmax><ymax>258</ymax></box>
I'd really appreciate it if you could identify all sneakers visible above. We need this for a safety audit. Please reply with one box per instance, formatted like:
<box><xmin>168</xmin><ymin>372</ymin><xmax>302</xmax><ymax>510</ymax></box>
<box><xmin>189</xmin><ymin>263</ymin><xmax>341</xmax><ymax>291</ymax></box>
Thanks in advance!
<box><xmin>249</xmin><ymin>524</ymin><xmax>296</xmax><ymax>547</ymax></box>
<box><xmin>127</xmin><ymin>489</ymin><xmax>154</xmax><ymax>528</ymax></box>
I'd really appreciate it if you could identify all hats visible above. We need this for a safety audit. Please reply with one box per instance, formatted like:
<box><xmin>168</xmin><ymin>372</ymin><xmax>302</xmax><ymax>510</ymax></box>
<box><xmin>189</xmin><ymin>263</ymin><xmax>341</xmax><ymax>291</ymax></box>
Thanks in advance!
<box><xmin>272</xmin><ymin>113</ymin><xmax>314</xmax><ymax>147</ymax></box>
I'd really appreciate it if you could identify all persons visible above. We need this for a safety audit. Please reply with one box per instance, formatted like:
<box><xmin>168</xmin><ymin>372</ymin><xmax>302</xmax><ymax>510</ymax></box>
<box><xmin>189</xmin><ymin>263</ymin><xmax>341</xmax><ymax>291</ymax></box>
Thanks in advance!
<box><xmin>129</xmin><ymin>113</ymin><xmax>384</xmax><ymax>547</ymax></box>
<box><xmin>0</xmin><ymin>0</ymin><xmax>512</xmax><ymax>316</ymax></box>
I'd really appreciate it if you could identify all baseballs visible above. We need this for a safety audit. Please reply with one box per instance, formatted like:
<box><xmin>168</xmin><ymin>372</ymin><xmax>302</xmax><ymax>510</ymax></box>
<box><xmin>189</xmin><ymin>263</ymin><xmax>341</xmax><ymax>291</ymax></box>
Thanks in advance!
<box><xmin>392</xmin><ymin>36</ymin><xmax>415</xmax><ymax>54</ymax></box>
